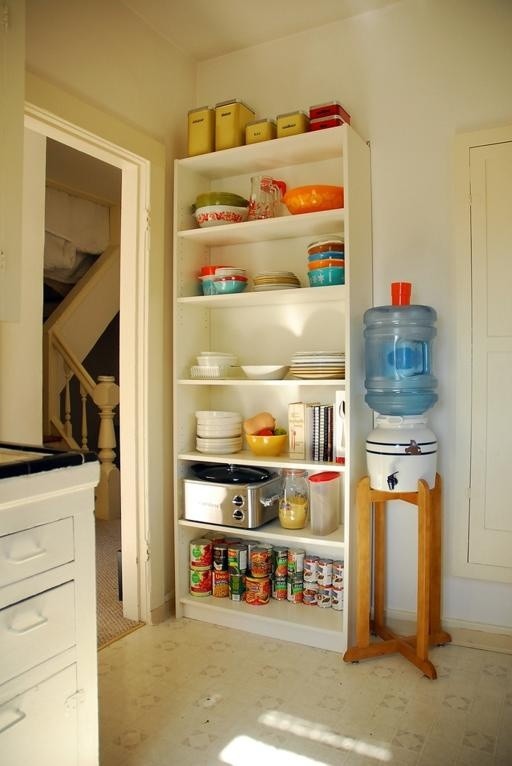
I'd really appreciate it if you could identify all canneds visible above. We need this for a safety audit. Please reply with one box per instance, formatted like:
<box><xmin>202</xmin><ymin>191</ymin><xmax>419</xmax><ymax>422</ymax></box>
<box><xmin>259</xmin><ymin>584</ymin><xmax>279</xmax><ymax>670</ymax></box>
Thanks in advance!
<box><xmin>189</xmin><ymin>533</ymin><xmax>344</xmax><ymax>612</ymax></box>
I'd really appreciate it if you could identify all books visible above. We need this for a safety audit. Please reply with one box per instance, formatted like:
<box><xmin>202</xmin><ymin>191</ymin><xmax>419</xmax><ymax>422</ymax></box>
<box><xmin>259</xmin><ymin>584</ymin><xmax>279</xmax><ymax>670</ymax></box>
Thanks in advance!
<box><xmin>306</xmin><ymin>406</ymin><xmax>335</xmax><ymax>463</ymax></box>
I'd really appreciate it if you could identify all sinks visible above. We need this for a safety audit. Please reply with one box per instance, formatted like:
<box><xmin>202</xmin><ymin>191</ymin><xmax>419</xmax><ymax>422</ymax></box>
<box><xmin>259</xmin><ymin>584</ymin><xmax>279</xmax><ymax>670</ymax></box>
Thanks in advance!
<box><xmin>1</xmin><ymin>441</ymin><xmax>51</xmax><ymax>465</ymax></box>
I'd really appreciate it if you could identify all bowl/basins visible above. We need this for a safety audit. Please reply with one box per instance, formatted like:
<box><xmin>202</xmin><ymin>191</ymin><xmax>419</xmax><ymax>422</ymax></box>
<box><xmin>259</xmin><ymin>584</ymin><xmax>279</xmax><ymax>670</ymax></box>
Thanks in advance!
<box><xmin>245</xmin><ymin>433</ymin><xmax>288</xmax><ymax>457</ymax></box>
<box><xmin>199</xmin><ymin>263</ymin><xmax>248</xmax><ymax>296</ymax></box>
<box><xmin>192</xmin><ymin>190</ymin><xmax>251</xmax><ymax>228</ymax></box>
<box><xmin>280</xmin><ymin>185</ymin><xmax>344</xmax><ymax>216</ymax></box>
<box><xmin>243</xmin><ymin>364</ymin><xmax>290</xmax><ymax>381</ymax></box>
<box><xmin>303</xmin><ymin>238</ymin><xmax>345</xmax><ymax>287</ymax></box>
<box><xmin>196</xmin><ymin>352</ymin><xmax>237</xmax><ymax>367</ymax></box>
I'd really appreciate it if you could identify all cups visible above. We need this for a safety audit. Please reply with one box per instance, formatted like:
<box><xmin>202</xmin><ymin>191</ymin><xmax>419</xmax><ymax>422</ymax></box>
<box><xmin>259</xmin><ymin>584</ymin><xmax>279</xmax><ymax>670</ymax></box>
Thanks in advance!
<box><xmin>245</xmin><ymin>175</ymin><xmax>288</xmax><ymax>221</ymax></box>
<box><xmin>391</xmin><ymin>282</ymin><xmax>412</xmax><ymax>305</ymax></box>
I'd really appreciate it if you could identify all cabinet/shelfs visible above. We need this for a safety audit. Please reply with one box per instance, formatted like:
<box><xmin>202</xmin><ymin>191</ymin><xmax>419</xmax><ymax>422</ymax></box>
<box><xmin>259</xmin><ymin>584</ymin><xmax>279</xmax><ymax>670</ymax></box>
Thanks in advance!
<box><xmin>161</xmin><ymin>111</ymin><xmax>379</xmax><ymax>658</ymax></box>
<box><xmin>340</xmin><ymin>475</ymin><xmax>453</xmax><ymax>680</ymax></box>
<box><xmin>1</xmin><ymin>460</ymin><xmax>111</xmax><ymax>766</ymax></box>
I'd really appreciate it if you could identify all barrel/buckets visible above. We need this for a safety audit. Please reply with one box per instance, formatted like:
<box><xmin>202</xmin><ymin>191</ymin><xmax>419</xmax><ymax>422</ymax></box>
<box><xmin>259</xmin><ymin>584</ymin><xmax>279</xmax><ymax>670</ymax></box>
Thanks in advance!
<box><xmin>363</xmin><ymin>304</ymin><xmax>438</xmax><ymax>415</ymax></box>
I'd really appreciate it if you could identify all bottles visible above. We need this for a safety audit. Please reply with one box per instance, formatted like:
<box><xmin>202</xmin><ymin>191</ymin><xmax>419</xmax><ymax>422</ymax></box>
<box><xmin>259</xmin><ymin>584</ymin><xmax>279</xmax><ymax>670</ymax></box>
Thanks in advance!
<box><xmin>278</xmin><ymin>469</ymin><xmax>309</xmax><ymax>529</ymax></box>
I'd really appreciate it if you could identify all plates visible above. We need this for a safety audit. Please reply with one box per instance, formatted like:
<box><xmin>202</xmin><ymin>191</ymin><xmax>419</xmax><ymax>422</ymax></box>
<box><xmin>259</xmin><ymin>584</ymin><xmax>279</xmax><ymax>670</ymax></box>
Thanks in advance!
<box><xmin>290</xmin><ymin>350</ymin><xmax>344</xmax><ymax>378</ymax></box>
<box><xmin>194</xmin><ymin>409</ymin><xmax>244</xmax><ymax>454</ymax></box>
<box><xmin>249</xmin><ymin>270</ymin><xmax>299</xmax><ymax>291</ymax></box>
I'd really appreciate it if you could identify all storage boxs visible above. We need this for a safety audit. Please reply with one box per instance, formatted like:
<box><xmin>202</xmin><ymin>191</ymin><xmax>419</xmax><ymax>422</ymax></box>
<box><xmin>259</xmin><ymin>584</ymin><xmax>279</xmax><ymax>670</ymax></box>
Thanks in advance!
<box><xmin>308</xmin><ymin>99</ymin><xmax>351</xmax><ymax>125</ymax></box>
<box><xmin>308</xmin><ymin>114</ymin><xmax>350</xmax><ymax>131</ymax></box>
<box><xmin>275</xmin><ymin>112</ymin><xmax>310</xmax><ymax>139</ymax></box>
<box><xmin>212</xmin><ymin>99</ymin><xmax>254</xmax><ymax>149</ymax></box>
<box><xmin>187</xmin><ymin>106</ymin><xmax>214</xmax><ymax>156</ymax></box>
<box><xmin>243</xmin><ymin>118</ymin><xmax>277</xmax><ymax>143</ymax></box>
